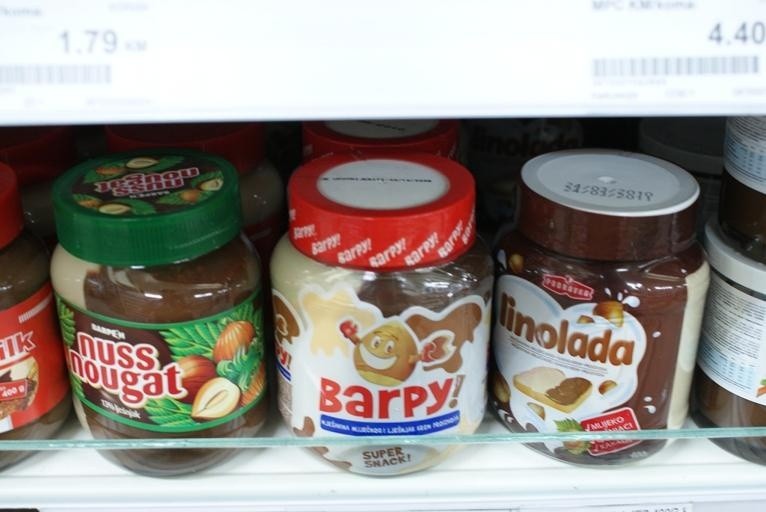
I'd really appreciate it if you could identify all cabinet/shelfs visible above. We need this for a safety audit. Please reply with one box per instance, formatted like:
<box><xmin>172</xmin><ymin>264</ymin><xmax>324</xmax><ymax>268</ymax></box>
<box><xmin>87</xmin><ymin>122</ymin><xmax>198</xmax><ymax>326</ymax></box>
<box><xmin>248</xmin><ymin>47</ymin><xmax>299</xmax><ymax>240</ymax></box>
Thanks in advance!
<box><xmin>0</xmin><ymin>415</ymin><xmax>766</xmax><ymax>512</ymax></box>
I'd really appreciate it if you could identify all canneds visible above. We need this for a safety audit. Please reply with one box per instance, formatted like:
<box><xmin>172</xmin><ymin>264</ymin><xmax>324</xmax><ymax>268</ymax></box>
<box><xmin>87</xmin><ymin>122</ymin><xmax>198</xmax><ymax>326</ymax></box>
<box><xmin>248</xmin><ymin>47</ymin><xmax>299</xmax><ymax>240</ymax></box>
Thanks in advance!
<box><xmin>0</xmin><ymin>116</ymin><xmax>765</xmax><ymax>476</ymax></box>
